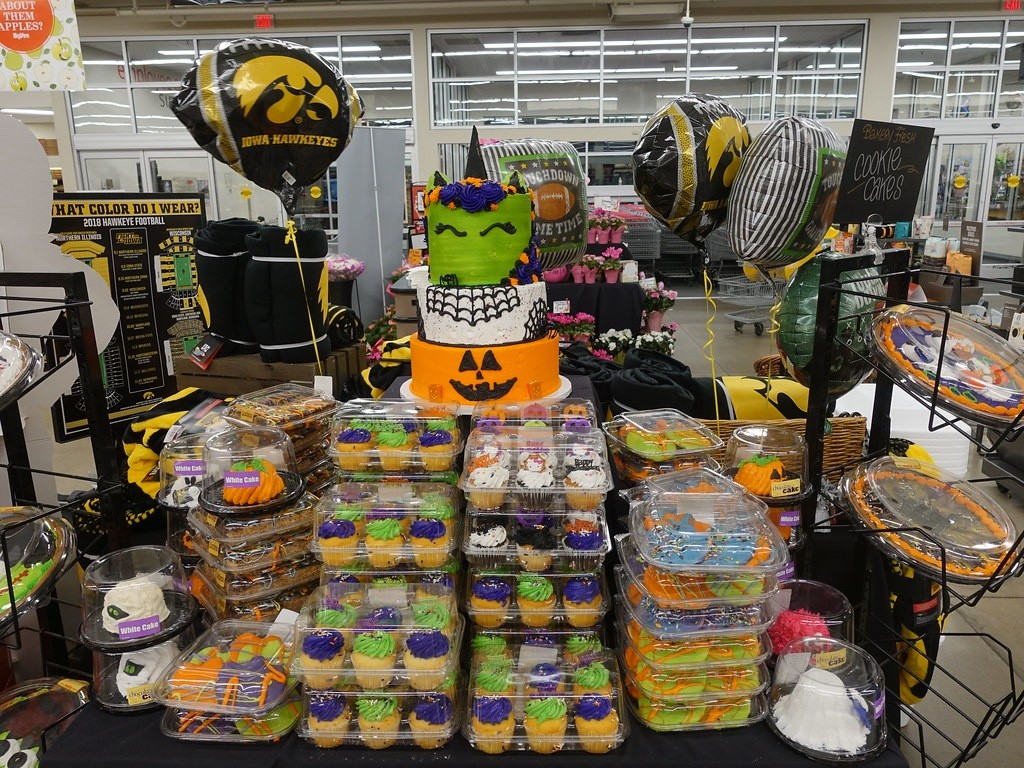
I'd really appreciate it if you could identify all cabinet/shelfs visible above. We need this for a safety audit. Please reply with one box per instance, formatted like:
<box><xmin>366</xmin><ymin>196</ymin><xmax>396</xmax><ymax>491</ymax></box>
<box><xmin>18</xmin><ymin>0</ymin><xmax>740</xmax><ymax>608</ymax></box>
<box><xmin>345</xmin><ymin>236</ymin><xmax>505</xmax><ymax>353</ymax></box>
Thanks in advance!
<box><xmin>0</xmin><ymin>259</ymin><xmax>141</xmax><ymax>768</ymax></box>
<box><xmin>796</xmin><ymin>244</ymin><xmax>1024</xmax><ymax>768</ymax></box>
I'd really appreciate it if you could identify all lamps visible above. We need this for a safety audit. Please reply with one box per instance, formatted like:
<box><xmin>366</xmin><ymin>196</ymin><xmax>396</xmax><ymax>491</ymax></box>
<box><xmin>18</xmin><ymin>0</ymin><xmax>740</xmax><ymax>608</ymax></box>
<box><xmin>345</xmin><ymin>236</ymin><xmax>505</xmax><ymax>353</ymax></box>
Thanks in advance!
<box><xmin>1</xmin><ymin>21</ymin><xmax>1023</xmax><ymax>134</ymax></box>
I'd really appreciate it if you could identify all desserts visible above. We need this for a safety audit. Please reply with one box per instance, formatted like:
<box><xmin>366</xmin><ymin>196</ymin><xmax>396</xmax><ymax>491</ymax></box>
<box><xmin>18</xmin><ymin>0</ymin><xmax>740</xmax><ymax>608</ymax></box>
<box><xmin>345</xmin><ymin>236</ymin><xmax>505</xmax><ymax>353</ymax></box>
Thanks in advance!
<box><xmin>300</xmin><ymin>415</ymin><xmax>619</xmax><ymax>754</ymax></box>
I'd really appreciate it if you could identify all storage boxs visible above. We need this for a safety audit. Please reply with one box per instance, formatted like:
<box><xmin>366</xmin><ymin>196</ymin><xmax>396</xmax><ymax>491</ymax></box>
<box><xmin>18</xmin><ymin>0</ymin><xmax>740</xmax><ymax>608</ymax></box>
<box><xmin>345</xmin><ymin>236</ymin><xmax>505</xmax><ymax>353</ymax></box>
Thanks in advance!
<box><xmin>149</xmin><ymin>382</ymin><xmax>795</xmax><ymax>755</ymax></box>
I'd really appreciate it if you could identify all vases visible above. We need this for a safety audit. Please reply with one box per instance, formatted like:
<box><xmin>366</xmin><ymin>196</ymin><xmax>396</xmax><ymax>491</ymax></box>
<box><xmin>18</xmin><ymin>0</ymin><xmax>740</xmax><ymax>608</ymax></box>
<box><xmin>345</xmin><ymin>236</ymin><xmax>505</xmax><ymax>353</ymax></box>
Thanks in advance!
<box><xmin>596</xmin><ymin>226</ymin><xmax>610</xmax><ymax>244</ymax></box>
<box><xmin>639</xmin><ymin>276</ymin><xmax>657</xmax><ymax>291</ymax></box>
<box><xmin>555</xmin><ymin>333</ymin><xmax>570</xmax><ymax>343</ymax></box>
<box><xmin>612</xmin><ymin>226</ymin><xmax>625</xmax><ymax>244</ymax></box>
<box><xmin>610</xmin><ymin>346</ymin><xmax>625</xmax><ymax>363</ymax></box>
<box><xmin>543</xmin><ymin>264</ymin><xmax>566</xmax><ymax>284</ymax></box>
<box><xmin>605</xmin><ymin>266</ymin><xmax>621</xmax><ymax>283</ymax></box>
<box><xmin>574</xmin><ymin>265</ymin><xmax>583</xmax><ymax>282</ymax></box>
<box><xmin>575</xmin><ymin>329</ymin><xmax>592</xmax><ymax>343</ymax></box>
<box><xmin>382</xmin><ymin>332</ymin><xmax>394</xmax><ymax>342</ymax></box>
<box><xmin>367</xmin><ymin>335</ymin><xmax>380</xmax><ymax>348</ymax></box>
<box><xmin>386</xmin><ymin>283</ymin><xmax>399</xmax><ymax>301</ymax></box>
<box><xmin>583</xmin><ymin>265</ymin><xmax>599</xmax><ymax>282</ymax></box>
<box><xmin>586</xmin><ymin>227</ymin><xmax>596</xmax><ymax>244</ymax></box>
<box><xmin>329</xmin><ymin>277</ymin><xmax>354</xmax><ymax>310</ymax></box>
<box><xmin>646</xmin><ymin>309</ymin><xmax>664</xmax><ymax>332</ymax></box>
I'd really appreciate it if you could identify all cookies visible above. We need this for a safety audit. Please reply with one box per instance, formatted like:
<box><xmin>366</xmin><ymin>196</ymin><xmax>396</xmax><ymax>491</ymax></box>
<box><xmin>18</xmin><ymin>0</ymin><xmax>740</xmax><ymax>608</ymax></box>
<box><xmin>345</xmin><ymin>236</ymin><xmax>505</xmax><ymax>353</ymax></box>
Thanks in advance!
<box><xmin>166</xmin><ymin>634</ymin><xmax>302</xmax><ymax>739</ymax></box>
<box><xmin>615</xmin><ymin>418</ymin><xmax>771</xmax><ymax>729</ymax></box>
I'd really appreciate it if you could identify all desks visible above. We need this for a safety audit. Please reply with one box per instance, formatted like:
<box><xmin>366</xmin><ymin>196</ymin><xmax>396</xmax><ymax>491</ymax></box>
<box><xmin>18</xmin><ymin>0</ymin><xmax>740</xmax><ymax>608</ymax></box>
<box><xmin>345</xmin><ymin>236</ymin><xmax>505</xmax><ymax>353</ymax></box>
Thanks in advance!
<box><xmin>39</xmin><ymin>617</ymin><xmax>908</xmax><ymax>768</ymax></box>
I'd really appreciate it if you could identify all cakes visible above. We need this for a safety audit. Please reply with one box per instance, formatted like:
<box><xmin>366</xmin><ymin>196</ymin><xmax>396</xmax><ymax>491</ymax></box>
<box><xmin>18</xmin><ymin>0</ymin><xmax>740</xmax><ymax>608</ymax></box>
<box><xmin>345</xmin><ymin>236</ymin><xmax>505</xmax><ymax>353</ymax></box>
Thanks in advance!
<box><xmin>165</xmin><ymin>464</ymin><xmax>224</xmax><ymax>508</ymax></box>
<box><xmin>409</xmin><ymin>124</ymin><xmax>561</xmax><ymax>407</ymax></box>
<box><xmin>223</xmin><ymin>459</ymin><xmax>284</xmax><ymax>505</ymax></box>
<box><xmin>0</xmin><ymin>509</ymin><xmax>64</xmax><ymax>620</ymax></box>
<box><xmin>771</xmin><ymin>667</ymin><xmax>873</xmax><ymax>753</ymax></box>
<box><xmin>733</xmin><ymin>453</ymin><xmax>792</xmax><ymax>541</ymax></box>
<box><xmin>0</xmin><ymin>689</ymin><xmax>81</xmax><ymax>768</ymax></box>
<box><xmin>852</xmin><ymin>470</ymin><xmax>1018</xmax><ymax>576</ymax></box>
<box><xmin>116</xmin><ymin>640</ymin><xmax>182</xmax><ymax>700</ymax></box>
<box><xmin>101</xmin><ymin>579</ymin><xmax>170</xmax><ymax>633</ymax></box>
<box><xmin>882</xmin><ymin>317</ymin><xmax>1024</xmax><ymax>417</ymax></box>
<box><xmin>767</xmin><ymin>607</ymin><xmax>833</xmax><ymax>661</ymax></box>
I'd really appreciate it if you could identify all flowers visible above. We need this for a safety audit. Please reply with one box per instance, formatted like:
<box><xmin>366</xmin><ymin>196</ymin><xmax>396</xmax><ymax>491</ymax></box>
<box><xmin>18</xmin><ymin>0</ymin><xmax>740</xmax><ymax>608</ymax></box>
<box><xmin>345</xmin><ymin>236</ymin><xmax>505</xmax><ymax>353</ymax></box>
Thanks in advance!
<box><xmin>325</xmin><ymin>209</ymin><xmax>678</xmax><ymax>365</ymax></box>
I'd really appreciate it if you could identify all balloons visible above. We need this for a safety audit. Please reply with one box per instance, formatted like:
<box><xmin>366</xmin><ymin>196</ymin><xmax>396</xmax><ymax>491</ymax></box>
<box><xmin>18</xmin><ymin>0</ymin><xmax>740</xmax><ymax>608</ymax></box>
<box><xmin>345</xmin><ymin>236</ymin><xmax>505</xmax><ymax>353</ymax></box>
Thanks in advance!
<box><xmin>482</xmin><ymin>141</ymin><xmax>589</xmax><ymax>274</ymax></box>
<box><xmin>170</xmin><ymin>36</ymin><xmax>366</xmax><ymax>209</ymax></box>
<box><xmin>631</xmin><ymin>92</ymin><xmax>888</xmax><ymax>398</ymax></box>
